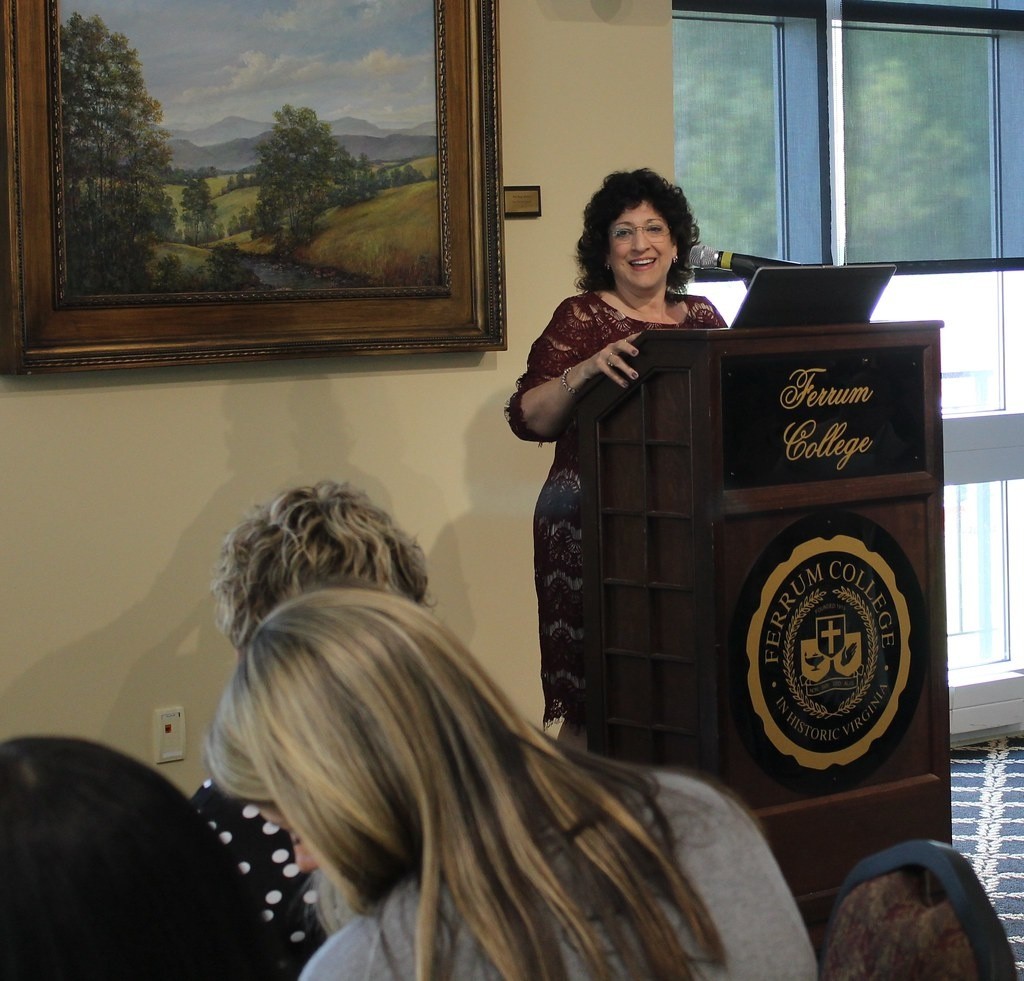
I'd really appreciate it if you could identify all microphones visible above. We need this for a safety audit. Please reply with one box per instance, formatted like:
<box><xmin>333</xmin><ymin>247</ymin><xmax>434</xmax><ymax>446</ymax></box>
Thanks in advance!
<box><xmin>689</xmin><ymin>246</ymin><xmax>799</xmax><ymax>279</ymax></box>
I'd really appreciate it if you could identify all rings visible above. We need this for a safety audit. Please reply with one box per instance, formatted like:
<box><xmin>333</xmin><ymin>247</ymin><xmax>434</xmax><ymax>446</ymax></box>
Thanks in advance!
<box><xmin>608</xmin><ymin>353</ymin><xmax>614</xmax><ymax>366</ymax></box>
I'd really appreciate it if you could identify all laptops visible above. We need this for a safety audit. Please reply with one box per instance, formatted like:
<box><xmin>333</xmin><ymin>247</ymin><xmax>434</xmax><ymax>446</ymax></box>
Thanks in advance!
<box><xmin>731</xmin><ymin>264</ymin><xmax>899</xmax><ymax>329</ymax></box>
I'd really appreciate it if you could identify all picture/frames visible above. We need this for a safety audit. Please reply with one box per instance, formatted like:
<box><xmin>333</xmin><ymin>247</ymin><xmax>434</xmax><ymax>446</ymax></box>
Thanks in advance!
<box><xmin>0</xmin><ymin>1</ymin><xmax>508</xmax><ymax>378</ymax></box>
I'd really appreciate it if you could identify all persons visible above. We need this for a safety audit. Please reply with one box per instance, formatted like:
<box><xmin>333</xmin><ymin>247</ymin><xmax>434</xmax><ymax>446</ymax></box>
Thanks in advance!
<box><xmin>190</xmin><ymin>481</ymin><xmax>428</xmax><ymax>981</ymax></box>
<box><xmin>206</xmin><ymin>585</ymin><xmax>819</xmax><ymax>981</ymax></box>
<box><xmin>503</xmin><ymin>171</ymin><xmax>727</xmax><ymax>753</ymax></box>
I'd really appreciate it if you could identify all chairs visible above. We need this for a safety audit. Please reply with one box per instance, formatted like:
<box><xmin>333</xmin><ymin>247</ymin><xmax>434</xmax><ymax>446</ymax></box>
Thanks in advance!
<box><xmin>816</xmin><ymin>840</ymin><xmax>1016</xmax><ymax>980</ymax></box>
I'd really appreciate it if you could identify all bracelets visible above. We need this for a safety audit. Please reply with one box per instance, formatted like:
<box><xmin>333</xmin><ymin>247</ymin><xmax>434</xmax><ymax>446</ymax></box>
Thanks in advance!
<box><xmin>561</xmin><ymin>366</ymin><xmax>581</xmax><ymax>393</ymax></box>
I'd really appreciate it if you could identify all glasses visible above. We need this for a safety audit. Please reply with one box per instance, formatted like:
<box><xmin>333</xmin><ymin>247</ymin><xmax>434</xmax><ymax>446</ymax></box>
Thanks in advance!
<box><xmin>608</xmin><ymin>222</ymin><xmax>672</xmax><ymax>240</ymax></box>
